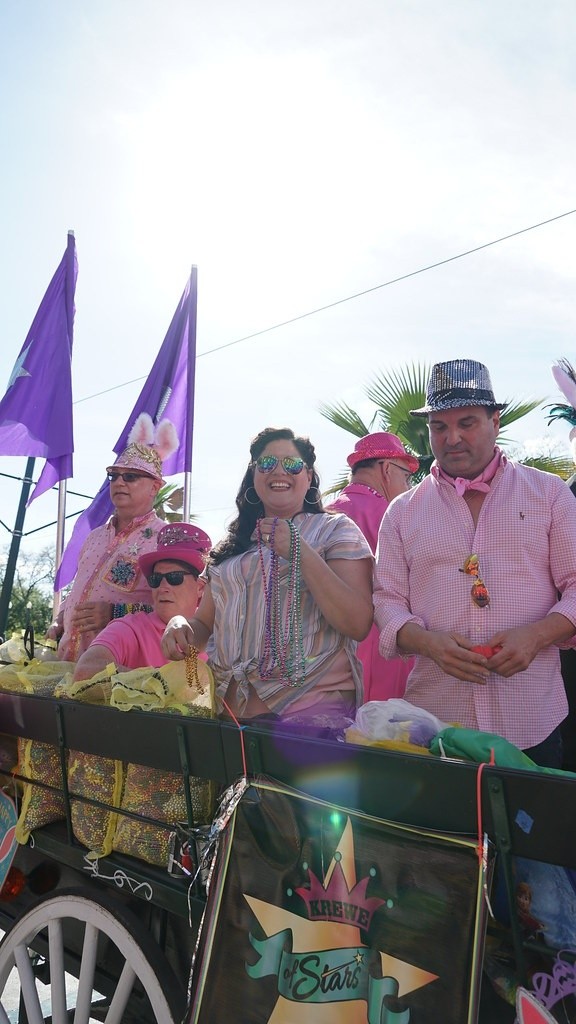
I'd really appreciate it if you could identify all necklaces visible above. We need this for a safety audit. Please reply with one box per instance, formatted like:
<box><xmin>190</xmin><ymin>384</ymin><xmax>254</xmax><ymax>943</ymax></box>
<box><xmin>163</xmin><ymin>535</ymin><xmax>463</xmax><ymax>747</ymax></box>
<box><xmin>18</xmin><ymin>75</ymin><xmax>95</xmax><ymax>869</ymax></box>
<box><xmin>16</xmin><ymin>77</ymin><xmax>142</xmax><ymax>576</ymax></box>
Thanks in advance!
<box><xmin>256</xmin><ymin>516</ymin><xmax>306</xmax><ymax>688</ymax></box>
<box><xmin>184</xmin><ymin>645</ymin><xmax>205</xmax><ymax>695</ymax></box>
<box><xmin>347</xmin><ymin>483</ymin><xmax>385</xmax><ymax>500</ymax></box>
<box><xmin>465</xmin><ymin>491</ymin><xmax>479</xmax><ymax>502</ymax></box>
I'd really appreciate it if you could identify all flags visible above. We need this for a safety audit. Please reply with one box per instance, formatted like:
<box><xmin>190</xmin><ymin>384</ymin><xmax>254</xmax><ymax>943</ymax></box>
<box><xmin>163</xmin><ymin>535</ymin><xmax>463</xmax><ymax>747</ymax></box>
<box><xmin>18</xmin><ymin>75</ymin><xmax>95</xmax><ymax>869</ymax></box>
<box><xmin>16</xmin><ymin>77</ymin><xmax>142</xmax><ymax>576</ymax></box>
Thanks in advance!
<box><xmin>1</xmin><ymin>234</ymin><xmax>79</xmax><ymax>509</ymax></box>
<box><xmin>54</xmin><ymin>268</ymin><xmax>197</xmax><ymax>593</ymax></box>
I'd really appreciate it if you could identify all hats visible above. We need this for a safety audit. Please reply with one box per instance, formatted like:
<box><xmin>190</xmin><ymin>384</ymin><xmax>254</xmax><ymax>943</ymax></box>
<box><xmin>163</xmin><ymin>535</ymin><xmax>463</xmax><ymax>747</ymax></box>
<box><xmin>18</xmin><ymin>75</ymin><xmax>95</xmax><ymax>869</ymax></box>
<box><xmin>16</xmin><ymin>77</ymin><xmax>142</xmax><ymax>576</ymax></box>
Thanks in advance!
<box><xmin>409</xmin><ymin>359</ymin><xmax>508</xmax><ymax>418</ymax></box>
<box><xmin>347</xmin><ymin>432</ymin><xmax>419</xmax><ymax>472</ymax></box>
<box><xmin>138</xmin><ymin>522</ymin><xmax>212</xmax><ymax>583</ymax></box>
<box><xmin>106</xmin><ymin>442</ymin><xmax>167</xmax><ymax>488</ymax></box>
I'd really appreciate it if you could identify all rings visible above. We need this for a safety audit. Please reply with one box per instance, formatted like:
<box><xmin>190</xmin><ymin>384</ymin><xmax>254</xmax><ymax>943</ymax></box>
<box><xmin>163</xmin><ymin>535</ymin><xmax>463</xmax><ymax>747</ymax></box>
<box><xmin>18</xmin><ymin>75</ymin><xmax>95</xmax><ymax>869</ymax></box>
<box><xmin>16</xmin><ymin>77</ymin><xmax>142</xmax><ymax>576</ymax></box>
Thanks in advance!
<box><xmin>267</xmin><ymin>535</ymin><xmax>270</xmax><ymax>541</ymax></box>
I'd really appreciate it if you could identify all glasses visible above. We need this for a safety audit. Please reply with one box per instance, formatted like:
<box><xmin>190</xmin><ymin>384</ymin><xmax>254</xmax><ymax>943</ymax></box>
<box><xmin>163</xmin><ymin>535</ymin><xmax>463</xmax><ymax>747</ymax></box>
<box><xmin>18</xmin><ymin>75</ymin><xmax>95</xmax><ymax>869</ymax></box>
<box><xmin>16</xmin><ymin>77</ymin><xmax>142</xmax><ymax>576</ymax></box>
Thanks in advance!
<box><xmin>252</xmin><ymin>455</ymin><xmax>308</xmax><ymax>476</ymax></box>
<box><xmin>146</xmin><ymin>571</ymin><xmax>194</xmax><ymax>588</ymax></box>
<box><xmin>379</xmin><ymin>461</ymin><xmax>415</xmax><ymax>485</ymax></box>
<box><xmin>107</xmin><ymin>471</ymin><xmax>156</xmax><ymax>482</ymax></box>
<box><xmin>458</xmin><ymin>553</ymin><xmax>491</xmax><ymax>610</ymax></box>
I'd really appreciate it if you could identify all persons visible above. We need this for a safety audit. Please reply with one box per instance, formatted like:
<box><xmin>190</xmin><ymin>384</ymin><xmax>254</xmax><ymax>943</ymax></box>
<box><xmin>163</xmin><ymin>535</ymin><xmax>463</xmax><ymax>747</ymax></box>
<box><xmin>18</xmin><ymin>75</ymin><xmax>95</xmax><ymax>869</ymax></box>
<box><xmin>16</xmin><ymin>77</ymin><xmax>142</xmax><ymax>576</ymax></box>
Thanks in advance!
<box><xmin>74</xmin><ymin>522</ymin><xmax>210</xmax><ymax>682</ymax></box>
<box><xmin>45</xmin><ymin>413</ymin><xmax>180</xmax><ymax>663</ymax></box>
<box><xmin>372</xmin><ymin>360</ymin><xmax>576</xmax><ymax>769</ymax></box>
<box><xmin>160</xmin><ymin>427</ymin><xmax>376</xmax><ymax>738</ymax></box>
<box><xmin>323</xmin><ymin>432</ymin><xmax>421</xmax><ymax>705</ymax></box>
